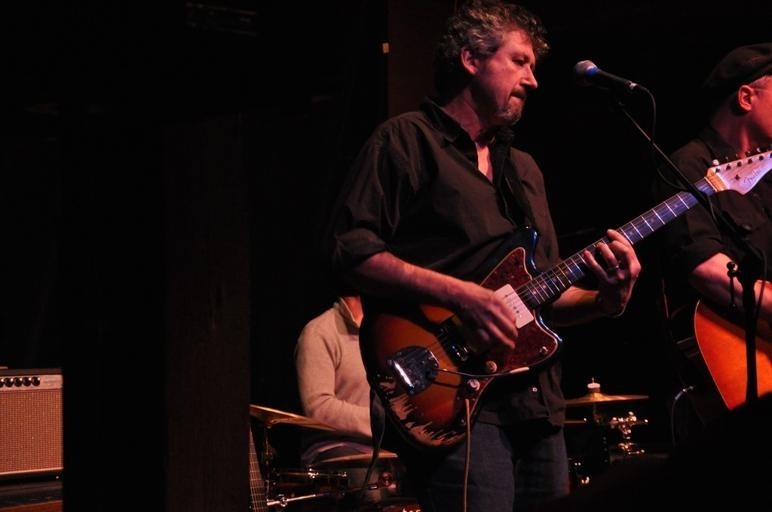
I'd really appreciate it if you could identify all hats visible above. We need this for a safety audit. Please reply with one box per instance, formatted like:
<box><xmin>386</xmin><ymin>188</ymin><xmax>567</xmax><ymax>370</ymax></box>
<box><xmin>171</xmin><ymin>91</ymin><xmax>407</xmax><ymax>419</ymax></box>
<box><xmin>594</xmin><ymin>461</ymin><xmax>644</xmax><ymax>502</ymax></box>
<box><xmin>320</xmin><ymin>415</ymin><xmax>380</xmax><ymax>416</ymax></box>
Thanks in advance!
<box><xmin>699</xmin><ymin>43</ymin><xmax>772</xmax><ymax>121</ymax></box>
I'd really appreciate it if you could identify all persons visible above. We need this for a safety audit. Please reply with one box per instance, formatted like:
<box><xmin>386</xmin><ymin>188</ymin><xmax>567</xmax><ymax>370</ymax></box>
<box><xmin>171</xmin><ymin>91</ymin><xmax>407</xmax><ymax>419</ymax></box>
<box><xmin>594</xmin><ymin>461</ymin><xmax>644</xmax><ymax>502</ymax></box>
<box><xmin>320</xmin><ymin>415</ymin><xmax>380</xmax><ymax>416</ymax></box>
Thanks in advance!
<box><xmin>327</xmin><ymin>0</ymin><xmax>641</xmax><ymax>512</ymax></box>
<box><xmin>294</xmin><ymin>295</ymin><xmax>381</xmax><ymax>503</ymax></box>
<box><xmin>657</xmin><ymin>46</ymin><xmax>772</xmax><ymax>342</ymax></box>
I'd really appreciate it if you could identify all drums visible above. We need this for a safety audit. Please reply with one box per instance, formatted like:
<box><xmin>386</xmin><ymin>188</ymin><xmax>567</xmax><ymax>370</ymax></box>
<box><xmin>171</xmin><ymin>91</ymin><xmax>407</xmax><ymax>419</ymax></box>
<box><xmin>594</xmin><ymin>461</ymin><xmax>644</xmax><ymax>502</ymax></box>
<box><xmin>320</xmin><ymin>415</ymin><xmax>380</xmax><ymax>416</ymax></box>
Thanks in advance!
<box><xmin>271</xmin><ymin>470</ymin><xmax>351</xmax><ymax>512</ymax></box>
<box><xmin>308</xmin><ymin>453</ymin><xmax>398</xmax><ymax>511</ymax></box>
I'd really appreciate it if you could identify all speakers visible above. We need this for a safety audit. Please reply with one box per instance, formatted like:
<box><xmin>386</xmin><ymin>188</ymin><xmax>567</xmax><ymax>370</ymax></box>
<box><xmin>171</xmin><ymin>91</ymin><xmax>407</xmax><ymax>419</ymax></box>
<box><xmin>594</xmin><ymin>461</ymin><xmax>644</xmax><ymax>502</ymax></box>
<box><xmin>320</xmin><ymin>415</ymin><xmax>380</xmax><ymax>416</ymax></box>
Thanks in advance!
<box><xmin>0</xmin><ymin>367</ymin><xmax>65</xmax><ymax>479</ymax></box>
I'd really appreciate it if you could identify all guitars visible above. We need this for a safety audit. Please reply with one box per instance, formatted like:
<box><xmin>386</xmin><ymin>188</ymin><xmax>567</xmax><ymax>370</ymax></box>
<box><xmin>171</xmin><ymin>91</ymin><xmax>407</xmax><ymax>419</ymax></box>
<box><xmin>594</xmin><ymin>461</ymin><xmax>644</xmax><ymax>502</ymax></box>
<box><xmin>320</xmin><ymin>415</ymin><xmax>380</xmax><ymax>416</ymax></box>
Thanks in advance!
<box><xmin>357</xmin><ymin>148</ymin><xmax>772</xmax><ymax>449</ymax></box>
<box><xmin>691</xmin><ymin>279</ymin><xmax>772</xmax><ymax>413</ymax></box>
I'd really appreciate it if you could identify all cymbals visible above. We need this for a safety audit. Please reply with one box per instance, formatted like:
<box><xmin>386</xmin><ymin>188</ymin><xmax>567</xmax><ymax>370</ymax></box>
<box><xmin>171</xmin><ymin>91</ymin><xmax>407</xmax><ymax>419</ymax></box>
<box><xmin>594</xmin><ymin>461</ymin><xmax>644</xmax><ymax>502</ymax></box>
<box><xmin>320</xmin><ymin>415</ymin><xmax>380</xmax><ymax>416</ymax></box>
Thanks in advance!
<box><xmin>249</xmin><ymin>404</ymin><xmax>337</xmax><ymax>436</ymax></box>
<box><xmin>563</xmin><ymin>390</ymin><xmax>649</xmax><ymax>405</ymax></box>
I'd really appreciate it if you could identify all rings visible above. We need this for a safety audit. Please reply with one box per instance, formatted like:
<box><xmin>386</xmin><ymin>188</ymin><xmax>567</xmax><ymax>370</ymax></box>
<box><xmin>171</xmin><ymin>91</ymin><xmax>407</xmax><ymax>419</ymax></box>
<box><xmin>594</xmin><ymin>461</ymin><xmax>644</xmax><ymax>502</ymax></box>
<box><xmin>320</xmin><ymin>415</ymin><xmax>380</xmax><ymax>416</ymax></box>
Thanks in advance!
<box><xmin>608</xmin><ymin>265</ymin><xmax>619</xmax><ymax>271</ymax></box>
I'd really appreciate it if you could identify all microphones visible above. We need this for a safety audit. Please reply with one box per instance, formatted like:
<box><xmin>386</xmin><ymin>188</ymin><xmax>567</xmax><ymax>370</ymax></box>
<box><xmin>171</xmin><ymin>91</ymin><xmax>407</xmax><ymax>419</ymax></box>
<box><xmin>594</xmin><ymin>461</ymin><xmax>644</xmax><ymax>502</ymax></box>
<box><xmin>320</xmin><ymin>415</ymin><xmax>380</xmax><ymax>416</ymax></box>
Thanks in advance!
<box><xmin>574</xmin><ymin>60</ymin><xmax>648</xmax><ymax>94</ymax></box>
